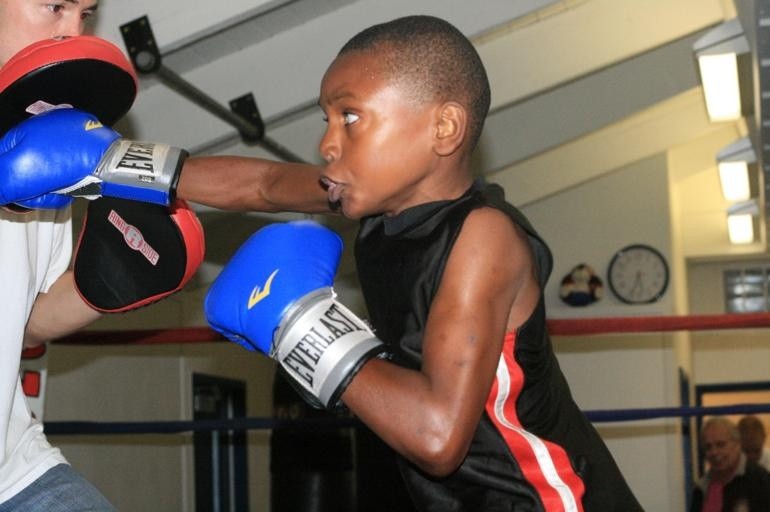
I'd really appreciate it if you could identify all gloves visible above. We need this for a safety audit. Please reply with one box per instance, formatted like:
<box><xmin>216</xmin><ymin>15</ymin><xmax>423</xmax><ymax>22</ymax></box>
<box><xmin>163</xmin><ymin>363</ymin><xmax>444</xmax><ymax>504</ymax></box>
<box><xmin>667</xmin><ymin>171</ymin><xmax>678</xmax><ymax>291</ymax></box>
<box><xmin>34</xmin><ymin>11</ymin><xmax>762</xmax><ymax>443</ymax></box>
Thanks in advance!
<box><xmin>0</xmin><ymin>108</ymin><xmax>189</xmax><ymax>214</ymax></box>
<box><xmin>205</xmin><ymin>218</ymin><xmax>390</xmax><ymax>413</ymax></box>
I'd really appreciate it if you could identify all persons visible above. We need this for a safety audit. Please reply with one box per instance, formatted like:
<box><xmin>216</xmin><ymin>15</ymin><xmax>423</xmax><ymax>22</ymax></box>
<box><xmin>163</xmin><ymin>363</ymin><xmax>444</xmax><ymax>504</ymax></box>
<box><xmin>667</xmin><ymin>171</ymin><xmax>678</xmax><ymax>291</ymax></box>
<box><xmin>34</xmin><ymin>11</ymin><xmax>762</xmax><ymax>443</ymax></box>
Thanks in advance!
<box><xmin>0</xmin><ymin>14</ymin><xmax>647</xmax><ymax>512</ymax></box>
<box><xmin>738</xmin><ymin>416</ymin><xmax>770</xmax><ymax>472</ymax></box>
<box><xmin>688</xmin><ymin>417</ymin><xmax>770</xmax><ymax>511</ymax></box>
<box><xmin>0</xmin><ymin>0</ymin><xmax>207</xmax><ymax>512</ymax></box>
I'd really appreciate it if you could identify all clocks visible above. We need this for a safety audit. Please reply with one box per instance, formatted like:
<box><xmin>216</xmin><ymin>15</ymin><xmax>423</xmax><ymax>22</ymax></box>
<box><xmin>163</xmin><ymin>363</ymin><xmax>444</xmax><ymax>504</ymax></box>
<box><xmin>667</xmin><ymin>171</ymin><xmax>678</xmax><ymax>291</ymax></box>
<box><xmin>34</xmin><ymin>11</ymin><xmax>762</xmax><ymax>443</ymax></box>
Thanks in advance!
<box><xmin>607</xmin><ymin>244</ymin><xmax>670</xmax><ymax>306</ymax></box>
